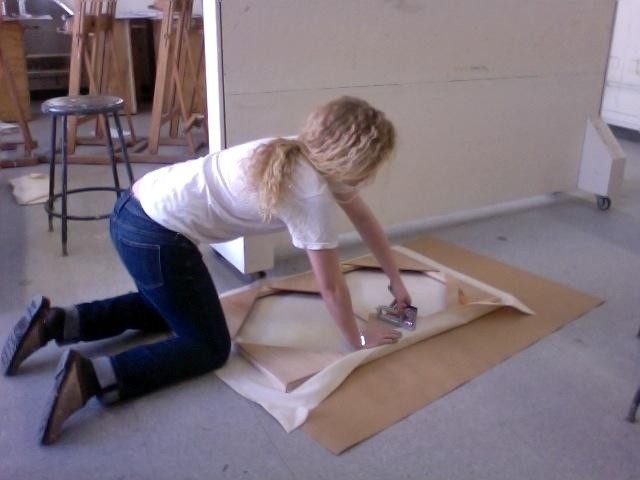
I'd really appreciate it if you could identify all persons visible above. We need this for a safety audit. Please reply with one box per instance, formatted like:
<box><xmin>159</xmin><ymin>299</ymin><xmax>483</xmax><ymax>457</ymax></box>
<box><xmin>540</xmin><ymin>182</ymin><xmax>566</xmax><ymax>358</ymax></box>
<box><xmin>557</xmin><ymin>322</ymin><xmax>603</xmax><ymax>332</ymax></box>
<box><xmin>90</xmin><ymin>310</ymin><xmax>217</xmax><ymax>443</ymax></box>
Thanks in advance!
<box><xmin>1</xmin><ymin>95</ymin><xmax>414</xmax><ymax>446</ymax></box>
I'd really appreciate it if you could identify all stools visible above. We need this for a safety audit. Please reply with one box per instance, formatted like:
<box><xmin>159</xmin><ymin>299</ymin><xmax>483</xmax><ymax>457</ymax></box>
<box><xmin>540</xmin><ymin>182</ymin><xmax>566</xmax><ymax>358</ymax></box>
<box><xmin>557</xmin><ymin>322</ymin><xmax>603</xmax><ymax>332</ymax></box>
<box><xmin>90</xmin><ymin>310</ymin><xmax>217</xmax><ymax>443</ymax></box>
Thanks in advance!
<box><xmin>41</xmin><ymin>94</ymin><xmax>135</xmax><ymax>258</ymax></box>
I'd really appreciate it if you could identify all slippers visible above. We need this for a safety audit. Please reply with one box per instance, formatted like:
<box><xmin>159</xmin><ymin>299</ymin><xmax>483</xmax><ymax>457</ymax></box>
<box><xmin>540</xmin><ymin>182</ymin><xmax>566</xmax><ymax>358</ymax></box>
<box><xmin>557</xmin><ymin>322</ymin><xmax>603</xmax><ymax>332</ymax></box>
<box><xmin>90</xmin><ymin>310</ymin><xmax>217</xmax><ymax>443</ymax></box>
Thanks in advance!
<box><xmin>39</xmin><ymin>349</ymin><xmax>88</xmax><ymax>445</ymax></box>
<box><xmin>1</xmin><ymin>295</ymin><xmax>50</xmax><ymax>376</ymax></box>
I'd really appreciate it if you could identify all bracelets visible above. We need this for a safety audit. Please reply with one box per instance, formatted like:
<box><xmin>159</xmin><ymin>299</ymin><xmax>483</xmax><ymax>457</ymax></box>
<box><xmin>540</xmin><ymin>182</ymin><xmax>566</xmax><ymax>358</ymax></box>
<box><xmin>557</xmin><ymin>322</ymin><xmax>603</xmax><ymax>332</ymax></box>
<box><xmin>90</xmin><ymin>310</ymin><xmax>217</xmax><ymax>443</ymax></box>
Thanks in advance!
<box><xmin>358</xmin><ymin>329</ymin><xmax>366</xmax><ymax>347</ymax></box>
